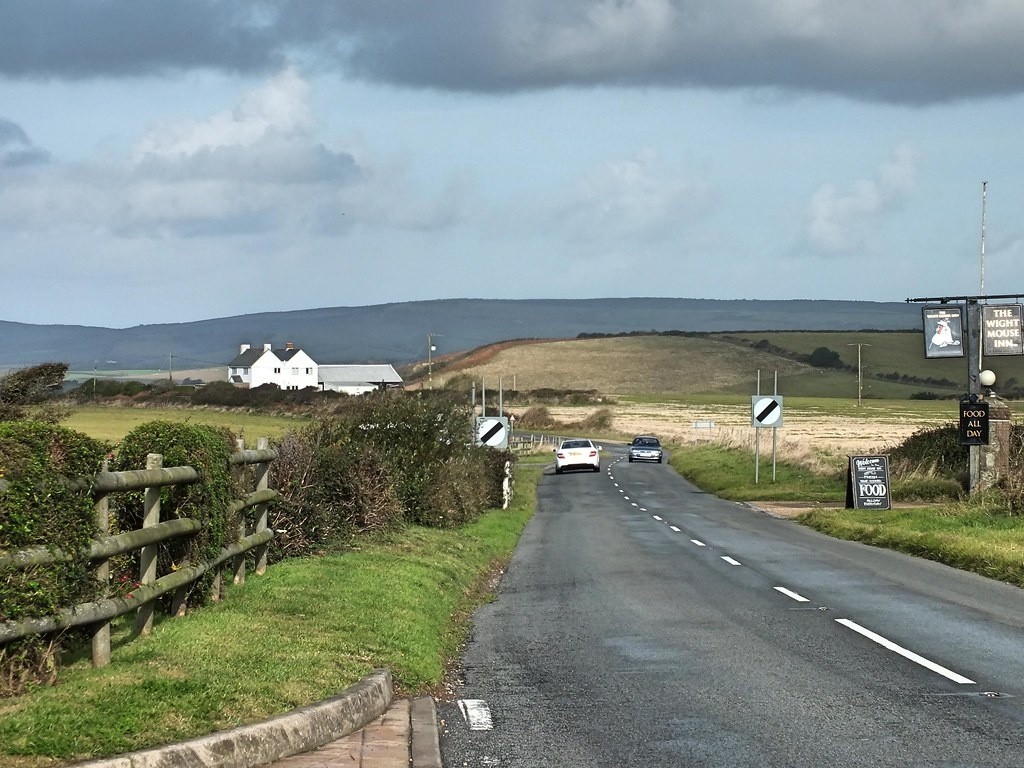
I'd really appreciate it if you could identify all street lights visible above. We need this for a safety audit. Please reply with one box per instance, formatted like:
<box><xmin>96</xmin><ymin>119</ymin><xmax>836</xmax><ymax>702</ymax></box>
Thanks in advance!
<box><xmin>426</xmin><ymin>332</ymin><xmax>437</xmax><ymax>391</ymax></box>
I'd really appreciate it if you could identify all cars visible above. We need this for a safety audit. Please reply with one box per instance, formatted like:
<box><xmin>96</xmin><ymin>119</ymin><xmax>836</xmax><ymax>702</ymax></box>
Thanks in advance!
<box><xmin>552</xmin><ymin>438</ymin><xmax>603</xmax><ymax>474</ymax></box>
<box><xmin>627</xmin><ymin>434</ymin><xmax>664</xmax><ymax>464</ymax></box>
